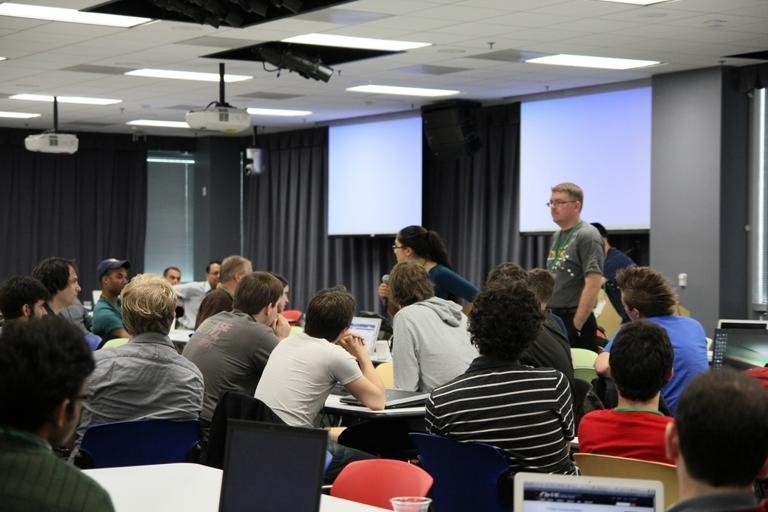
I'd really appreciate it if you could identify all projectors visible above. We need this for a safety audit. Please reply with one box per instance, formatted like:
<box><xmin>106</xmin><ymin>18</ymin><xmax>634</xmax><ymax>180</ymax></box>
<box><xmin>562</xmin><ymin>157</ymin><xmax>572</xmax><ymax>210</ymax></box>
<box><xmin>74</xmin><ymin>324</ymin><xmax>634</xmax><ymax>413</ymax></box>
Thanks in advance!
<box><xmin>186</xmin><ymin>107</ymin><xmax>250</xmax><ymax>132</ymax></box>
<box><xmin>24</xmin><ymin>134</ymin><xmax>79</xmax><ymax>154</ymax></box>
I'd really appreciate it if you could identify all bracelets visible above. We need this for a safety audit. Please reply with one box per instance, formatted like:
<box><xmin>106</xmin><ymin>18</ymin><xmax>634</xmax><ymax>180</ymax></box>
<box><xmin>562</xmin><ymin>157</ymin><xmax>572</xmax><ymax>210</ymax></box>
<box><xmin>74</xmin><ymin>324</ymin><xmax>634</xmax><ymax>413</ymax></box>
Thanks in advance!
<box><xmin>575</xmin><ymin>326</ymin><xmax>583</xmax><ymax>333</ymax></box>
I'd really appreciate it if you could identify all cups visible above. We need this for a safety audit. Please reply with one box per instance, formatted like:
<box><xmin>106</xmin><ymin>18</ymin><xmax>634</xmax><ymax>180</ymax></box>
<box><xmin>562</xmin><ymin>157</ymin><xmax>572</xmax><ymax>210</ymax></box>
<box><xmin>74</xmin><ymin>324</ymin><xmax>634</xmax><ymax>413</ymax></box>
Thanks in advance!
<box><xmin>374</xmin><ymin>340</ymin><xmax>391</xmax><ymax>360</ymax></box>
<box><xmin>389</xmin><ymin>497</ymin><xmax>431</xmax><ymax>511</ymax></box>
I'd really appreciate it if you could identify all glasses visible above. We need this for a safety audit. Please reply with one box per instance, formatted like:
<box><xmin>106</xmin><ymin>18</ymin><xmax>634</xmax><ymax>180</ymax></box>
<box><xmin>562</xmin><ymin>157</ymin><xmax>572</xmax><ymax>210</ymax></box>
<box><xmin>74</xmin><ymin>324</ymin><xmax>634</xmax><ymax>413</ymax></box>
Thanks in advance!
<box><xmin>546</xmin><ymin>200</ymin><xmax>576</xmax><ymax>206</ymax></box>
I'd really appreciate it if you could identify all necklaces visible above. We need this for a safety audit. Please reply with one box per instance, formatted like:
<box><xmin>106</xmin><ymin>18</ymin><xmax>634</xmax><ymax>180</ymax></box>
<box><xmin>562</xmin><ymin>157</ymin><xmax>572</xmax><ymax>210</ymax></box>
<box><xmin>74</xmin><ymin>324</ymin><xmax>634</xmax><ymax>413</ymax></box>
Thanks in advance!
<box><xmin>419</xmin><ymin>258</ymin><xmax>430</xmax><ymax>268</ymax></box>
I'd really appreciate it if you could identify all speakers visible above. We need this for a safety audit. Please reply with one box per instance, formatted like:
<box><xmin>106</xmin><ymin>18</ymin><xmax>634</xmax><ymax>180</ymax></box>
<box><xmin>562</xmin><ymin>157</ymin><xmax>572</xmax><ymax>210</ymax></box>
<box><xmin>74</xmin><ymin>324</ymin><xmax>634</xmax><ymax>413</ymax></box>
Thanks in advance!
<box><xmin>244</xmin><ymin>148</ymin><xmax>263</xmax><ymax>177</ymax></box>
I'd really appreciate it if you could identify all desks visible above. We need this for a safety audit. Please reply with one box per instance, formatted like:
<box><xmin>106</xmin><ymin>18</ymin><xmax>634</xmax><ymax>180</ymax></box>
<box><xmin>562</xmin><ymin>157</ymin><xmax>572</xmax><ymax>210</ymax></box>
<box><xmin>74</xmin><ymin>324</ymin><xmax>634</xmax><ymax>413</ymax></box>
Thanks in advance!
<box><xmin>321</xmin><ymin>389</ymin><xmax>431</xmax><ymax>420</ymax></box>
<box><xmin>79</xmin><ymin>463</ymin><xmax>392</xmax><ymax>509</ymax></box>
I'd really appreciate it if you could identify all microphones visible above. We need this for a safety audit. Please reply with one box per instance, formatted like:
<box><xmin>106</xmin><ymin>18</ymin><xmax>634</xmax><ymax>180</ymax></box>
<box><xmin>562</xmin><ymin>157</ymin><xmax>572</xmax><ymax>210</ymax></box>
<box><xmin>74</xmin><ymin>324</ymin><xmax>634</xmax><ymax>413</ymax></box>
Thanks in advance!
<box><xmin>381</xmin><ymin>274</ymin><xmax>390</xmax><ymax>304</ymax></box>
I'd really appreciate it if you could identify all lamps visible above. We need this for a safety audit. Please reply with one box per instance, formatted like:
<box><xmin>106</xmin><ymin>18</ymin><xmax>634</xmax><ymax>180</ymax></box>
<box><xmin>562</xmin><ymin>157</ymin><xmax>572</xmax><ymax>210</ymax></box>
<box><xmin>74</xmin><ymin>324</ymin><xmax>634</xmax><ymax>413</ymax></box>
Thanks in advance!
<box><xmin>155</xmin><ymin>0</ymin><xmax>307</xmax><ymax>32</ymax></box>
<box><xmin>253</xmin><ymin>41</ymin><xmax>335</xmax><ymax>85</ymax></box>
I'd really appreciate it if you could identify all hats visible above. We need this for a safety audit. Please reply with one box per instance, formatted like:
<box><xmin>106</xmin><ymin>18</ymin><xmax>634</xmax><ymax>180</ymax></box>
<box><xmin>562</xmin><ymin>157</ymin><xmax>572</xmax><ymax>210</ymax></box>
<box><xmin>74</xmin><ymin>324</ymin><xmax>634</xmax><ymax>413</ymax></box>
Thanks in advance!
<box><xmin>96</xmin><ymin>258</ymin><xmax>130</xmax><ymax>279</ymax></box>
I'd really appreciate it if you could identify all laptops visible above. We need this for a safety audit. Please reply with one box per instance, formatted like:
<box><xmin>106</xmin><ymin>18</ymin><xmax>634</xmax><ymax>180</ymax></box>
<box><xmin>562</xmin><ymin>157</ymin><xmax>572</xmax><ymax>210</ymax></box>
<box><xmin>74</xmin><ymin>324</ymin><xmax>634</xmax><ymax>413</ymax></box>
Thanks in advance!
<box><xmin>339</xmin><ymin>388</ymin><xmax>432</xmax><ymax>409</ymax></box>
<box><xmin>712</xmin><ymin>328</ymin><xmax>768</xmax><ymax>373</ymax></box>
<box><xmin>513</xmin><ymin>472</ymin><xmax>664</xmax><ymax>512</ymax></box>
<box><xmin>218</xmin><ymin>418</ymin><xmax>329</xmax><ymax>512</ymax></box>
<box><xmin>707</xmin><ymin>319</ymin><xmax>768</xmax><ymax>362</ymax></box>
<box><xmin>346</xmin><ymin>316</ymin><xmax>383</xmax><ymax>352</ymax></box>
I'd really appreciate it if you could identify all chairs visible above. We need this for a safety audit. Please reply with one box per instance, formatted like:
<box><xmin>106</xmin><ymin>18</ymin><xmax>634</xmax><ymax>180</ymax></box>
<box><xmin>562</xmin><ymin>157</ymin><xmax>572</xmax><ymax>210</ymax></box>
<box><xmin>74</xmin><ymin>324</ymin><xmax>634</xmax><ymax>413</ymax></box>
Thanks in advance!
<box><xmin>408</xmin><ymin>431</ymin><xmax>536</xmax><ymax>510</ymax></box>
<box><xmin>572</xmin><ymin>453</ymin><xmax>680</xmax><ymax>511</ymax></box>
<box><xmin>75</xmin><ymin>418</ymin><xmax>203</xmax><ymax>470</ymax></box>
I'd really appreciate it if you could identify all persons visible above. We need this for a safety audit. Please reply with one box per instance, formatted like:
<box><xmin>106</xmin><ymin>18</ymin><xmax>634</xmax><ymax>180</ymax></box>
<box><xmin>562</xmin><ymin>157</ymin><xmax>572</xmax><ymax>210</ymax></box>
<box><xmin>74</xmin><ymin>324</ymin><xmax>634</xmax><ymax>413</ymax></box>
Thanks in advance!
<box><xmin>589</xmin><ymin>222</ymin><xmax>637</xmax><ymax>324</ymax></box>
<box><xmin>1</xmin><ymin>315</ymin><xmax>114</xmax><ymax>512</ymax></box>
<box><xmin>1</xmin><ymin>255</ymin><xmax>385</xmax><ymax>486</ymax></box>
<box><xmin>389</xmin><ymin>261</ymin><xmax>768</xmax><ymax>512</ymax></box>
<box><xmin>378</xmin><ymin>226</ymin><xmax>482</xmax><ymax>315</ymax></box>
<box><xmin>541</xmin><ymin>182</ymin><xmax>604</xmax><ymax>353</ymax></box>
